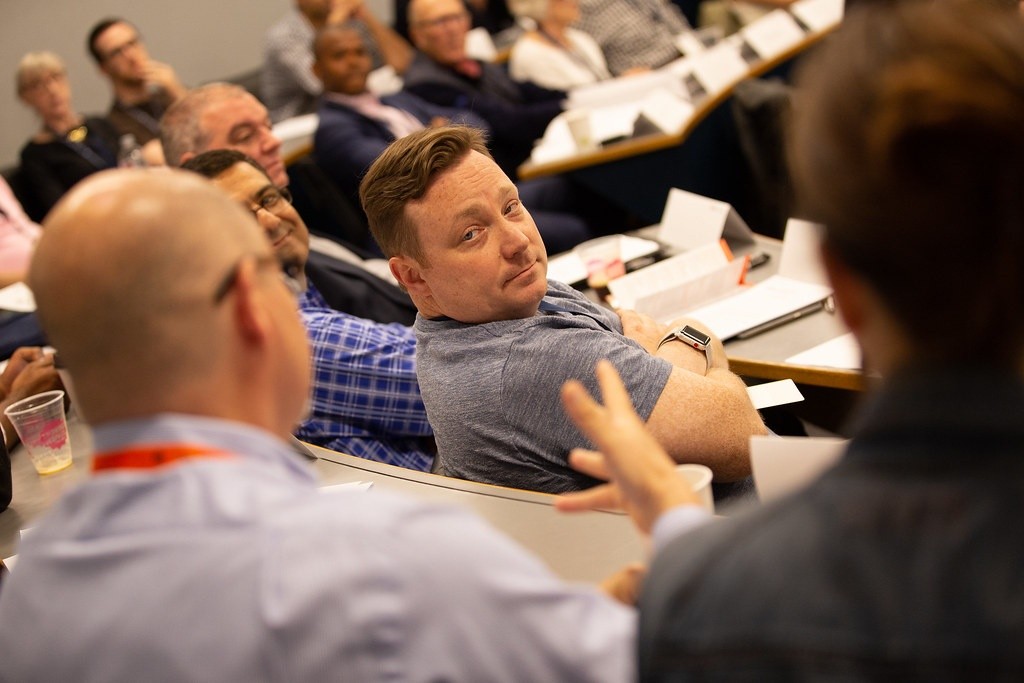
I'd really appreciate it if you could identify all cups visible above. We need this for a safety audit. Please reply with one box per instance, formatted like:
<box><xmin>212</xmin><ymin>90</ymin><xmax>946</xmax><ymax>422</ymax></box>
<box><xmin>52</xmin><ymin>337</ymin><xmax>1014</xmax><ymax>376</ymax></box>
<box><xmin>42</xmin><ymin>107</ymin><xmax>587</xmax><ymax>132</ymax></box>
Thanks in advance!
<box><xmin>676</xmin><ymin>464</ymin><xmax>713</xmax><ymax>515</ymax></box>
<box><xmin>566</xmin><ymin>116</ymin><xmax>591</xmax><ymax>152</ymax></box>
<box><xmin>574</xmin><ymin>234</ymin><xmax>627</xmax><ymax>291</ymax></box>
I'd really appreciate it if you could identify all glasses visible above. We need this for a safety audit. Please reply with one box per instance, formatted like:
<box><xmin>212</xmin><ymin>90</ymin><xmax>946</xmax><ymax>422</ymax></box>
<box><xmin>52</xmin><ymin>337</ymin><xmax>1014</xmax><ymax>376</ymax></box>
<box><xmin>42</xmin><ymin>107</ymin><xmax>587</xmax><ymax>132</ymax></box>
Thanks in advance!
<box><xmin>248</xmin><ymin>187</ymin><xmax>292</xmax><ymax>223</ymax></box>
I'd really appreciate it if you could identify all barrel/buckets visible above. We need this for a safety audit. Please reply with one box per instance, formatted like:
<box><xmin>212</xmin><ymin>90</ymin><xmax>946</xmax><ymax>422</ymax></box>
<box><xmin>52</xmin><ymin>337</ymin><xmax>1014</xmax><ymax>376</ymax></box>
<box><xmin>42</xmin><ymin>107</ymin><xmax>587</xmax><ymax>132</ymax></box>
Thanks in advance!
<box><xmin>4</xmin><ymin>390</ymin><xmax>71</xmax><ymax>475</ymax></box>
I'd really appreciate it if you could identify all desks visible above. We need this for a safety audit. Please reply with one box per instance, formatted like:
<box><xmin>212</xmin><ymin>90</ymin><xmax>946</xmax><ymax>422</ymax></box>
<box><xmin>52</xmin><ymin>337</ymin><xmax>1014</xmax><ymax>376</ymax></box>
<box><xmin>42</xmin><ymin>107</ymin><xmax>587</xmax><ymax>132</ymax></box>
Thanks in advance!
<box><xmin>0</xmin><ymin>0</ymin><xmax>864</xmax><ymax>592</ymax></box>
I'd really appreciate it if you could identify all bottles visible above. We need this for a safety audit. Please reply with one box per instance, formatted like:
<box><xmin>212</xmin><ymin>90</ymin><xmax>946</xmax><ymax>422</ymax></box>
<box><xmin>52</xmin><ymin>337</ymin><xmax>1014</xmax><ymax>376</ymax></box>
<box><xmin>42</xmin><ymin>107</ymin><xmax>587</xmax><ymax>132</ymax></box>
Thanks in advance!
<box><xmin>116</xmin><ymin>133</ymin><xmax>143</xmax><ymax>165</ymax></box>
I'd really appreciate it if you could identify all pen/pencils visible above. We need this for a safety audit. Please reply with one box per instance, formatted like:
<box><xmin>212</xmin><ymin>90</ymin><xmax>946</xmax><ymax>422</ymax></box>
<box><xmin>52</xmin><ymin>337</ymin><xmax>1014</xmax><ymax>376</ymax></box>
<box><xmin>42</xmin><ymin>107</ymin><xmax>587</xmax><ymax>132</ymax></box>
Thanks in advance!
<box><xmin>747</xmin><ymin>252</ymin><xmax>770</xmax><ymax>269</ymax></box>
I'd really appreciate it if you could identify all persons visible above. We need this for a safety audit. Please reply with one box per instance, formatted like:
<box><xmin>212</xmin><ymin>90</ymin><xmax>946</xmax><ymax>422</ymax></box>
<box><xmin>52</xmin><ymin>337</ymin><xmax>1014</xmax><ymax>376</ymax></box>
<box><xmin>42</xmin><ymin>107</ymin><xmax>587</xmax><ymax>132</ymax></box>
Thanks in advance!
<box><xmin>701</xmin><ymin>0</ymin><xmax>796</xmax><ymax>38</ymax></box>
<box><xmin>634</xmin><ymin>0</ymin><xmax>1023</xmax><ymax>683</ymax></box>
<box><xmin>183</xmin><ymin>149</ymin><xmax>441</xmax><ymax>474</ymax></box>
<box><xmin>9</xmin><ymin>51</ymin><xmax>166</xmax><ymax>225</ymax></box>
<box><xmin>158</xmin><ymin>82</ymin><xmax>416</xmax><ymax>326</ymax></box>
<box><xmin>312</xmin><ymin>29</ymin><xmax>451</xmax><ymax>254</ymax></box>
<box><xmin>1</xmin><ymin>345</ymin><xmax>70</xmax><ymax>510</ymax></box>
<box><xmin>260</xmin><ymin>0</ymin><xmax>414</xmax><ymax>123</ymax></box>
<box><xmin>404</xmin><ymin>0</ymin><xmax>693</xmax><ymax>184</ymax></box>
<box><xmin>87</xmin><ymin>18</ymin><xmax>185</xmax><ymax>147</ymax></box>
<box><xmin>358</xmin><ymin>124</ymin><xmax>770</xmax><ymax>498</ymax></box>
<box><xmin>1</xmin><ymin>166</ymin><xmax>710</xmax><ymax>683</ymax></box>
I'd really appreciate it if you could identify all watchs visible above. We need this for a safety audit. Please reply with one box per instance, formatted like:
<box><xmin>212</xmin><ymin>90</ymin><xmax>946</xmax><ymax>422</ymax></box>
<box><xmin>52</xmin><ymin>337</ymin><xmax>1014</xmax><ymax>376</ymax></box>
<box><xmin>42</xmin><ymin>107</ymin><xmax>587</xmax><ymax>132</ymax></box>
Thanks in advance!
<box><xmin>657</xmin><ymin>324</ymin><xmax>713</xmax><ymax>374</ymax></box>
